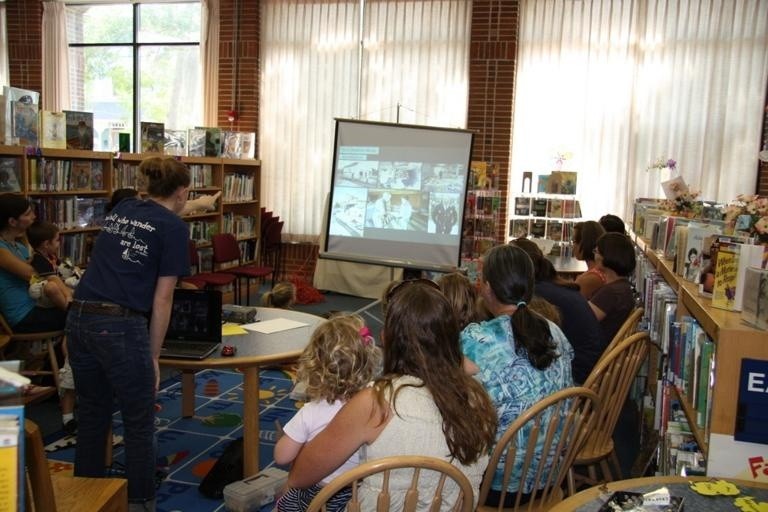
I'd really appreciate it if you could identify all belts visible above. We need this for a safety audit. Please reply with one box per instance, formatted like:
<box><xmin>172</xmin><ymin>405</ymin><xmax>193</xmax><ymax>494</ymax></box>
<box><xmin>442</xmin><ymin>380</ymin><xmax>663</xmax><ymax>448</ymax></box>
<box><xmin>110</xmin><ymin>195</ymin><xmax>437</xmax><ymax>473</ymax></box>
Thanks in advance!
<box><xmin>63</xmin><ymin>299</ymin><xmax>140</xmax><ymax>319</ymax></box>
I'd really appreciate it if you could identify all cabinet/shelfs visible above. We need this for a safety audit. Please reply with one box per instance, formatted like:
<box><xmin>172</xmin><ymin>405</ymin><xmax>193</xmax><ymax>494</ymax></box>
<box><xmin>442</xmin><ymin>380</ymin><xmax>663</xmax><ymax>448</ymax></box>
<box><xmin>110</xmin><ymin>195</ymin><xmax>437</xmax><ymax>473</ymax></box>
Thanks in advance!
<box><xmin>221</xmin><ymin>159</ymin><xmax>263</xmax><ymax>303</ymax></box>
<box><xmin>625</xmin><ymin>223</ymin><xmax>767</xmax><ymax>485</ymax></box>
<box><xmin>113</xmin><ymin>152</ymin><xmax>224</xmax><ymax>276</ymax></box>
<box><xmin>27</xmin><ymin>148</ymin><xmax>113</xmax><ymax>270</ymax></box>
<box><xmin>0</xmin><ymin>146</ymin><xmax>30</xmax><ymax>256</ymax></box>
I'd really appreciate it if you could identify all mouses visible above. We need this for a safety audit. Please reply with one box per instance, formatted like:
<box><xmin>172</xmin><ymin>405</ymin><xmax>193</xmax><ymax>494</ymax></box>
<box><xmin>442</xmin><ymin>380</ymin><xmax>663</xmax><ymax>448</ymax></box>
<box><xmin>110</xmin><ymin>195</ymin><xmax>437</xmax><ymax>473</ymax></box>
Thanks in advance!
<box><xmin>223</xmin><ymin>344</ymin><xmax>236</xmax><ymax>355</ymax></box>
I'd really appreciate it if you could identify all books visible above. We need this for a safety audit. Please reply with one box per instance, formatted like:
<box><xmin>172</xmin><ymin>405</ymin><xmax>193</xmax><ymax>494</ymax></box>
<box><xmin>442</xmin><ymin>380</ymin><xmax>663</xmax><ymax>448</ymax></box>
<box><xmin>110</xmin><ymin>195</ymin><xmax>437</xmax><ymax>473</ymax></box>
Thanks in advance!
<box><xmin>634</xmin><ymin>248</ymin><xmax>715</xmax><ymax>477</ymax></box>
<box><xmin>633</xmin><ymin>198</ymin><xmax>767</xmax><ymax>329</ymax></box>
<box><xmin>597</xmin><ymin>489</ymin><xmax>686</xmax><ymax>512</ymax></box>
<box><xmin>426</xmin><ymin>161</ymin><xmax>584</xmax><ymax>258</ymax></box>
<box><xmin>0</xmin><ymin>86</ymin><xmax>93</xmax><ymax>150</ymax></box>
<box><xmin>186</xmin><ymin>163</ymin><xmax>214</xmax><ymax>273</ymax></box>
<box><xmin>32</xmin><ymin>197</ymin><xmax>106</xmax><ymax>267</ymax></box>
<box><xmin>140</xmin><ymin>122</ymin><xmax>255</xmax><ymax>160</ymax></box>
<box><xmin>222</xmin><ymin>172</ymin><xmax>258</xmax><ymax>267</ymax></box>
<box><xmin>1</xmin><ymin>157</ymin><xmax>104</xmax><ymax>192</ymax></box>
<box><xmin>0</xmin><ymin>359</ymin><xmax>32</xmax><ymax>512</ymax></box>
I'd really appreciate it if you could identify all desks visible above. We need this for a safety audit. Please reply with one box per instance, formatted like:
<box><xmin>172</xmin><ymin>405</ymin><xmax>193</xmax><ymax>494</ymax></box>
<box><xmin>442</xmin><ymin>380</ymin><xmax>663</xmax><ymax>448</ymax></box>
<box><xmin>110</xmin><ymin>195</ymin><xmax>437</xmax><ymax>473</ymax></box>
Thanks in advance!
<box><xmin>548</xmin><ymin>475</ymin><xmax>768</xmax><ymax>512</ymax></box>
<box><xmin>0</xmin><ymin>475</ymin><xmax>129</xmax><ymax>511</ymax></box>
<box><xmin>104</xmin><ymin>305</ymin><xmax>330</xmax><ymax>481</ymax></box>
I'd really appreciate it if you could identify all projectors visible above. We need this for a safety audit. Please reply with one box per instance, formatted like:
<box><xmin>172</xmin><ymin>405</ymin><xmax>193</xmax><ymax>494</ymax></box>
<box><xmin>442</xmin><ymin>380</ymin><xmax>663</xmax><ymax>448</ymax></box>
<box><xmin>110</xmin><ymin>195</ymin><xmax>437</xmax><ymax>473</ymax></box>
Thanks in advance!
<box><xmin>222</xmin><ymin>304</ymin><xmax>257</xmax><ymax>324</ymax></box>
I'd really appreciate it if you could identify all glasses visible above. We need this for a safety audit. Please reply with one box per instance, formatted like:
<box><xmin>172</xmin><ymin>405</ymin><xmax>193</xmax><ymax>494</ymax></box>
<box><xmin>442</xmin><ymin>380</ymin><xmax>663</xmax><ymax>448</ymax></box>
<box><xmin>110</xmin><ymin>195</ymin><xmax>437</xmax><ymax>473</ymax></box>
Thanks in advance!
<box><xmin>386</xmin><ymin>278</ymin><xmax>441</xmax><ymax>301</ymax></box>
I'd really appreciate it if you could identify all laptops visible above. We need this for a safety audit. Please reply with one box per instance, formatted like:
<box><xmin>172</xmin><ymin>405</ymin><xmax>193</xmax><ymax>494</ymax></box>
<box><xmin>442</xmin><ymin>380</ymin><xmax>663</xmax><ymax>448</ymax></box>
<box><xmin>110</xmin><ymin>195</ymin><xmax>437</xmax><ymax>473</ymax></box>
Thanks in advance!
<box><xmin>161</xmin><ymin>289</ymin><xmax>222</xmax><ymax>361</ymax></box>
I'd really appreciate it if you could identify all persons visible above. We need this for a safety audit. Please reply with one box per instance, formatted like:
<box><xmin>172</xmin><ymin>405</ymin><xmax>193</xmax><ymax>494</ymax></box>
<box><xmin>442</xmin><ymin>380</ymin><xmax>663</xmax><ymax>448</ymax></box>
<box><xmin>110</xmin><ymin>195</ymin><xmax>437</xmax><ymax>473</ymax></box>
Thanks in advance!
<box><xmin>440</xmin><ymin>238</ymin><xmax>604</xmax><ymax>509</ymax></box>
<box><xmin>28</xmin><ymin>221</ymin><xmax>80</xmax><ymax>314</ymax></box>
<box><xmin>391</xmin><ymin>195</ymin><xmax>414</xmax><ymax>229</ymax></box>
<box><xmin>288</xmin><ymin>281</ymin><xmax>497</xmax><ymax>512</ymax></box>
<box><xmin>274</xmin><ymin>316</ymin><xmax>382</xmax><ymax>510</ymax></box>
<box><xmin>370</xmin><ymin>192</ymin><xmax>392</xmax><ymax>230</ymax></box>
<box><xmin>260</xmin><ymin>282</ymin><xmax>297</xmax><ymax>311</ymax></box>
<box><xmin>105</xmin><ymin>188</ymin><xmax>141</xmax><ymax>214</ymax></box>
<box><xmin>572</xmin><ymin>215</ymin><xmax>636</xmax><ymax>346</ymax></box>
<box><xmin>0</xmin><ymin>193</ymin><xmax>77</xmax><ymax>432</ymax></box>
<box><xmin>64</xmin><ymin>156</ymin><xmax>222</xmax><ymax>503</ymax></box>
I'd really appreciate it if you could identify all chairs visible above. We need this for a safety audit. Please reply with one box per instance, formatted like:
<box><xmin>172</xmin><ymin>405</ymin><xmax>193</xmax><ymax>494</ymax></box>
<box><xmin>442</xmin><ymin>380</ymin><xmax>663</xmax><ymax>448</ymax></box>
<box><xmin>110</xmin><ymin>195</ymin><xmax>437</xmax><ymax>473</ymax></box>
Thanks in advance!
<box><xmin>211</xmin><ymin>233</ymin><xmax>274</xmax><ymax>307</ymax></box>
<box><xmin>578</xmin><ymin>307</ymin><xmax>648</xmax><ymax>476</ymax></box>
<box><xmin>0</xmin><ymin>315</ymin><xmax>64</xmax><ymax>398</ymax></box>
<box><xmin>475</xmin><ymin>385</ymin><xmax>603</xmax><ymax>511</ymax></box>
<box><xmin>308</xmin><ymin>455</ymin><xmax>473</xmax><ymax>512</ymax></box>
<box><xmin>187</xmin><ymin>240</ymin><xmax>238</xmax><ymax>305</ymax></box>
<box><xmin>562</xmin><ymin>331</ymin><xmax>652</xmax><ymax>496</ymax></box>
<box><xmin>178</xmin><ymin>278</ymin><xmax>207</xmax><ymax>291</ymax></box>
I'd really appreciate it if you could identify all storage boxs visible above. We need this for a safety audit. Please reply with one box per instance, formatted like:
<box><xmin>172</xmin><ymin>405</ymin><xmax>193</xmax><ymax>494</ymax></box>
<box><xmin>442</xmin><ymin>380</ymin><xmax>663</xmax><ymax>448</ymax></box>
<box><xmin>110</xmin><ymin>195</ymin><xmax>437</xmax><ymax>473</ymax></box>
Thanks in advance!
<box><xmin>222</xmin><ymin>467</ymin><xmax>290</xmax><ymax>511</ymax></box>
